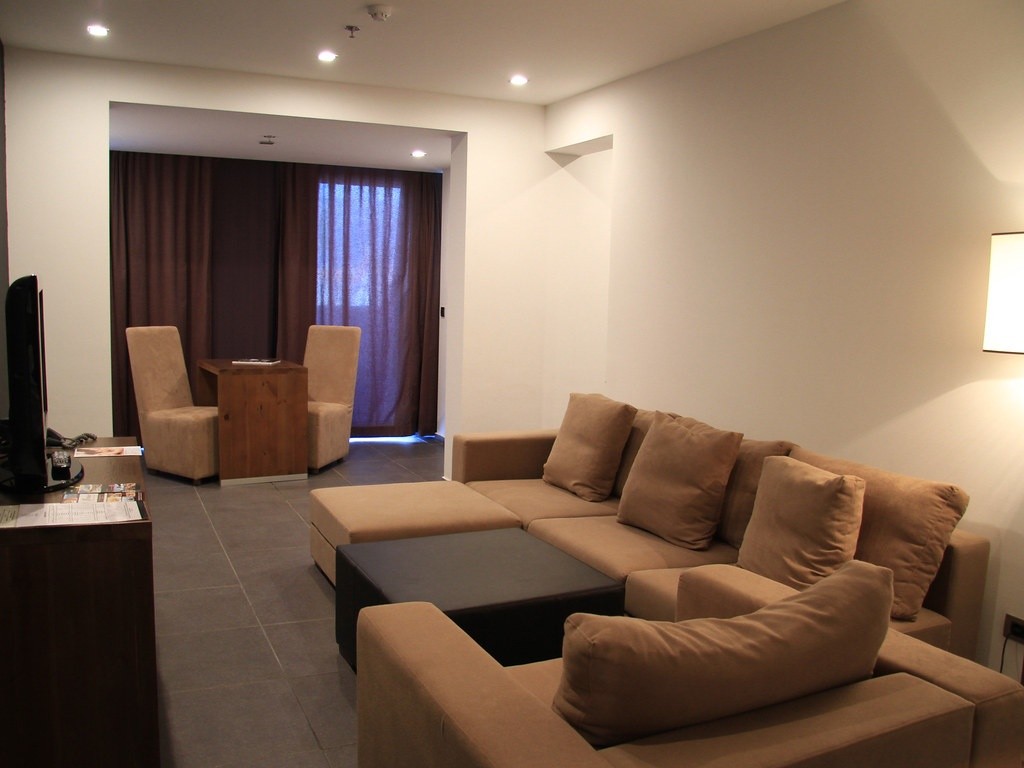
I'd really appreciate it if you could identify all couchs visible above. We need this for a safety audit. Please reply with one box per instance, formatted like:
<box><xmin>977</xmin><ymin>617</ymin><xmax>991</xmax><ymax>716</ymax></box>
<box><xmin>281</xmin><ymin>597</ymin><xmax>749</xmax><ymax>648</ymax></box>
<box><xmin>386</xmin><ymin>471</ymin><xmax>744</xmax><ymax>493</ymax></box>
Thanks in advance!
<box><xmin>357</xmin><ymin>563</ymin><xmax>1024</xmax><ymax>768</ymax></box>
<box><xmin>451</xmin><ymin>431</ymin><xmax>990</xmax><ymax>664</ymax></box>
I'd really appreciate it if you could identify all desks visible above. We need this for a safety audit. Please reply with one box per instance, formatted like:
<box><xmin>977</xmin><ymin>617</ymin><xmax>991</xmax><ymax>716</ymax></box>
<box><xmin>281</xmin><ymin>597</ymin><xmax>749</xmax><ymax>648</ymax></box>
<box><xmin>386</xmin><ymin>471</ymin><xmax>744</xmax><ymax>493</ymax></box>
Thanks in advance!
<box><xmin>0</xmin><ymin>436</ymin><xmax>161</xmax><ymax>768</ymax></box>
<box><xmin>197</xmin><ymin>356</ymin><xmax>308</xmax><ymax>486</ymax></box>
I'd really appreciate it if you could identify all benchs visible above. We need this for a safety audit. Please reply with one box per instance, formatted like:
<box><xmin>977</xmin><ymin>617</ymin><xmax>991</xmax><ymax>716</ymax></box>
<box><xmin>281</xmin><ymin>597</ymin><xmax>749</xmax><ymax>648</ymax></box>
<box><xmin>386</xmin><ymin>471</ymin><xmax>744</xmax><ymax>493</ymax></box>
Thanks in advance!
<box><xmin>310</xmin><ymin>480</ymin><xmax>522</xmax><ymax>588</ymax></box>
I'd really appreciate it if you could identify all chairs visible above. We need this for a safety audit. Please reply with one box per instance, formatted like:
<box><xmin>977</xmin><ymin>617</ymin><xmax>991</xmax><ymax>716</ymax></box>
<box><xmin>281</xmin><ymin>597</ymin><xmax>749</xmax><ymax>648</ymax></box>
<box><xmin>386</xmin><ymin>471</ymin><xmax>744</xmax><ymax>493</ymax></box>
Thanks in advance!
<box><xmin>126</xmin><ymin>326</ymin><xmax>218</xmax><ymax>485</ymax></box>
<box><xmin>303</xmin><ymin>325</ymin><xmax>361</xmax><ymax>474</ymax></box>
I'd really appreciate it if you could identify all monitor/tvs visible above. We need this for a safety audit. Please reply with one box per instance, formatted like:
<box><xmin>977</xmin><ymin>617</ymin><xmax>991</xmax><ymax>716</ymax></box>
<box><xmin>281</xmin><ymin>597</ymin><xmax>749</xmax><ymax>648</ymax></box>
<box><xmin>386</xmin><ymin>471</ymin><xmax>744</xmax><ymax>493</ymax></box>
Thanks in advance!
<box><xmin>0</xmin><ymin>275</ymin><xmax>84</xmax><ymax>494</ymax></box>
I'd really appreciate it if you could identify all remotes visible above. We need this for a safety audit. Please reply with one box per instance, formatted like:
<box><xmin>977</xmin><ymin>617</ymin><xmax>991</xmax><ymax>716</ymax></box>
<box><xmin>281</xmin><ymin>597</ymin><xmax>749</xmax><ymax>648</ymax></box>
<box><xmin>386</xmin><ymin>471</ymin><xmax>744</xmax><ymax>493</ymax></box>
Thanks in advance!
<box><xmin>52</xmin><ymin>450</ymin><xmax>71</xmax><ymax>469</ymax></box>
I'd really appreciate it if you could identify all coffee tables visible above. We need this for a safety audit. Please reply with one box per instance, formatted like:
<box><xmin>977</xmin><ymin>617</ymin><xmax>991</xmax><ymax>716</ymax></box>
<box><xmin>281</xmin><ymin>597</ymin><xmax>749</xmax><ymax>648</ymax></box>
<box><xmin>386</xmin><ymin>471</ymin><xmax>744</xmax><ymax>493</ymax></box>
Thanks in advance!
<box><xmin>336</xmin><ymin>528</ymin><xmax>625</xmax><ymax>674</ymax></box>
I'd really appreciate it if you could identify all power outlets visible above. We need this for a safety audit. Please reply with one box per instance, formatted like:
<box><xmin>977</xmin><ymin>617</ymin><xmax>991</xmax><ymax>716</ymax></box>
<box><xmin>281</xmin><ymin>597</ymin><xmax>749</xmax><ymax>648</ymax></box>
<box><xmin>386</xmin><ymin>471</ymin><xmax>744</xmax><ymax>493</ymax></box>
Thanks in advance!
<box><xmin>1003</xmin><ymin>613</ymin><xmax>1024</xmax><ymax>645</ymax></box>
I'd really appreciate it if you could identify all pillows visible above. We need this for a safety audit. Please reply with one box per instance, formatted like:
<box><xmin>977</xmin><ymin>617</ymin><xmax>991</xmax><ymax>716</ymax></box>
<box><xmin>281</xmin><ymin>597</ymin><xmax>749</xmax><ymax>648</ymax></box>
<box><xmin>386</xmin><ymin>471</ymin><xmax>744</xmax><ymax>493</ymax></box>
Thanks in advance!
<box><xmin>551</xmin><ymin>558</ymin><xmax>895</xmax><ymax>749</ymax></box>
<box><xmin>617</xmin><ymin>411</ymin><xmax>744</xmax><ymax>550</ymax></box>
<box><xmin>612</xmin><ymin>410</ymin><xmax>681</xmax><ymax>498</ymax></box>
<box><xmin>788</xmin><ymin>447</ymin><xmax>970</xmax><ymax>621</ymax></box>
<box><xmin>675</xmin><ymin>417</ymin><xmax>800</xmax><ymax>548</ymax></box>
<box><xmin>543</xmin><ymin>393</ymin><xmax>638</xmax><ymax>501</ymax></box>
<box><xmin>737</xmin><ymin>456</ymin><xmax>866</xmax><ymax>593</ymax></box>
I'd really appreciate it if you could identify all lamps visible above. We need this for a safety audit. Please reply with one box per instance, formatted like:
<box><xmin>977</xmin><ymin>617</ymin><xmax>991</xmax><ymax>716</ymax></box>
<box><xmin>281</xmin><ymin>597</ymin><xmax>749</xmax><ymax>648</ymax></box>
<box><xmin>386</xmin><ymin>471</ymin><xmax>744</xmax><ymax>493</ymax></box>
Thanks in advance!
<box><xmin>981</xmin><ymin>230</ymin><xmax>1024</xmax><ymax>355</ymax></box>
